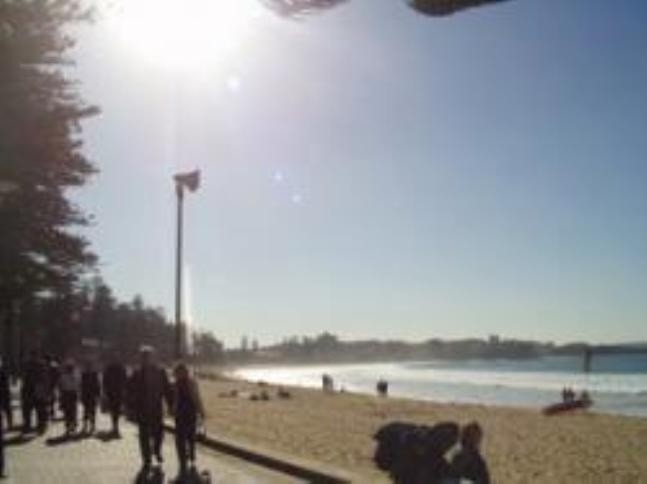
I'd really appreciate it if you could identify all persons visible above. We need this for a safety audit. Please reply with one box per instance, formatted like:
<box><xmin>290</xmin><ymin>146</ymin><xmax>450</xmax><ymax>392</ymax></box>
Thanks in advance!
<box><xmin>452</xmin><ymin>419</ymin><xmax>491</xmax><ymax>484</ymax></box>
<box><xmin>392</xmin><ymin>421</ymin><xmax>459</xmax><ymax>483</ymax></box>
<box><xmin>0</xmin><ymin>348</ymin><xmax>128</xmax><ymax>439</ymax></box>
<box><xmin>127</xmin><ymin>343</ymin><xmax>171</xmax><ymax>467</ymax></box>
<box><xmin>166</xmin><ymin>361</ymin><xmax>207</xmax><ymax>476</ymax></box>
